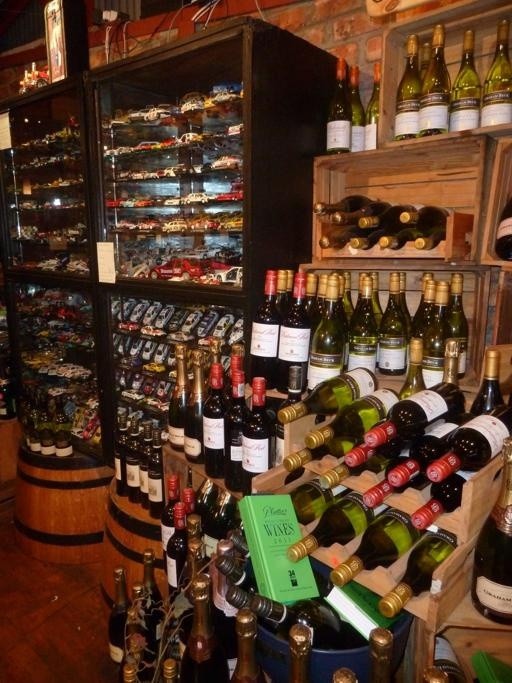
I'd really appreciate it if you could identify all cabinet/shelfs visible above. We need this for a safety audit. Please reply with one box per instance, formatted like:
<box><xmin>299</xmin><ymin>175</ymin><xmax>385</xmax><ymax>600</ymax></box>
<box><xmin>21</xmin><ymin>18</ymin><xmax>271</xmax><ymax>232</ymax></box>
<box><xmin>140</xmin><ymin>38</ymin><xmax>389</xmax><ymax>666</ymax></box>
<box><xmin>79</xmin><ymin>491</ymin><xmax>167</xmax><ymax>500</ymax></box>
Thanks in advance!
<box><xmin>163</xmin><ymin>0</ymin><xmax>511</xmax><ymax>682</ymax></box>
<box><xmin>1</xmin><ymin>75</ymin><xmax>109</xmax><ymax>464</ymax></box>
<box><xmin>89</xmin><ymin>18</ymin><xmax>351</xmax><ymax>463</ymax></box>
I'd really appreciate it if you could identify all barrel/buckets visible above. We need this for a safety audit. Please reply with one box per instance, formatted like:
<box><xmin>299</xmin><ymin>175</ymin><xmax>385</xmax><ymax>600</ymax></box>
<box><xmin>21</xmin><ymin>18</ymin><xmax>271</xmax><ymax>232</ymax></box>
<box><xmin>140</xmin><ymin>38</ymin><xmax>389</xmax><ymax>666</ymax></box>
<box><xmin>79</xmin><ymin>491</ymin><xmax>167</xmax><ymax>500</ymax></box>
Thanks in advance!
<box><xmin>238</xmin><ymin>554</ymin><xmax>413</xmax><ymax>682</ymax></box>
<box><xmin>99</xmin><ymin>476</ymin><xmax>172</xmax><ymax>614</ymax></box>
<box><xmin>15</xmin><ymin>440</ymin><xmax>116</xmax><ymax>566</ymax></box>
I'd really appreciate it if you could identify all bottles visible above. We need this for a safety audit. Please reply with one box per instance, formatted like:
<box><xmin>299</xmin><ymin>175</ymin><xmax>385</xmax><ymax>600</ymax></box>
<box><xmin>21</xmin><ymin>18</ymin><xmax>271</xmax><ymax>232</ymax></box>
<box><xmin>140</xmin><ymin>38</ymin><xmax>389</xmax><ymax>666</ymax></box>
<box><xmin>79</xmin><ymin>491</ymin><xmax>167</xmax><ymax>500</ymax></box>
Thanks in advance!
<box><xmin>106</xmin><ymin>550</ymin><xmax>164</xmax><ymax>680</ymax></box>
<box><xmin>17</xmin><ymin>396</ymin><xmax>74</xmax><ymax>461</ymax></box>
<box><xmin>288</xmin><ymin>500</ymin><xmax>453</xmax><ymax>624</ymax></box>
<box><xmin>165</xmin><ymin>503</ymin><xmax>226</xmax><ymax>678</ymax></box>
<box><xmin>486</xmin><ymin>186</ymin><xmax>510</xmax><ymax>262</ymax></box>
<box><xmin>311</xmin><ymin>193</ymin><xmax>474</xmax><ymax>252</ymax></box>
<box><xmin>303</xmin><ymin>373</ymin><xmax>510</xmax><ymax>514</ymax></box>
<box><xmin>322</xmin><ymin>13</ymin><xmax>512</xmax><ymax>153</ymax></box>
<box><xmin>172</xmin><ymin>348</ymin><xmax>298</xmax><ymax>487</ymax></box>
